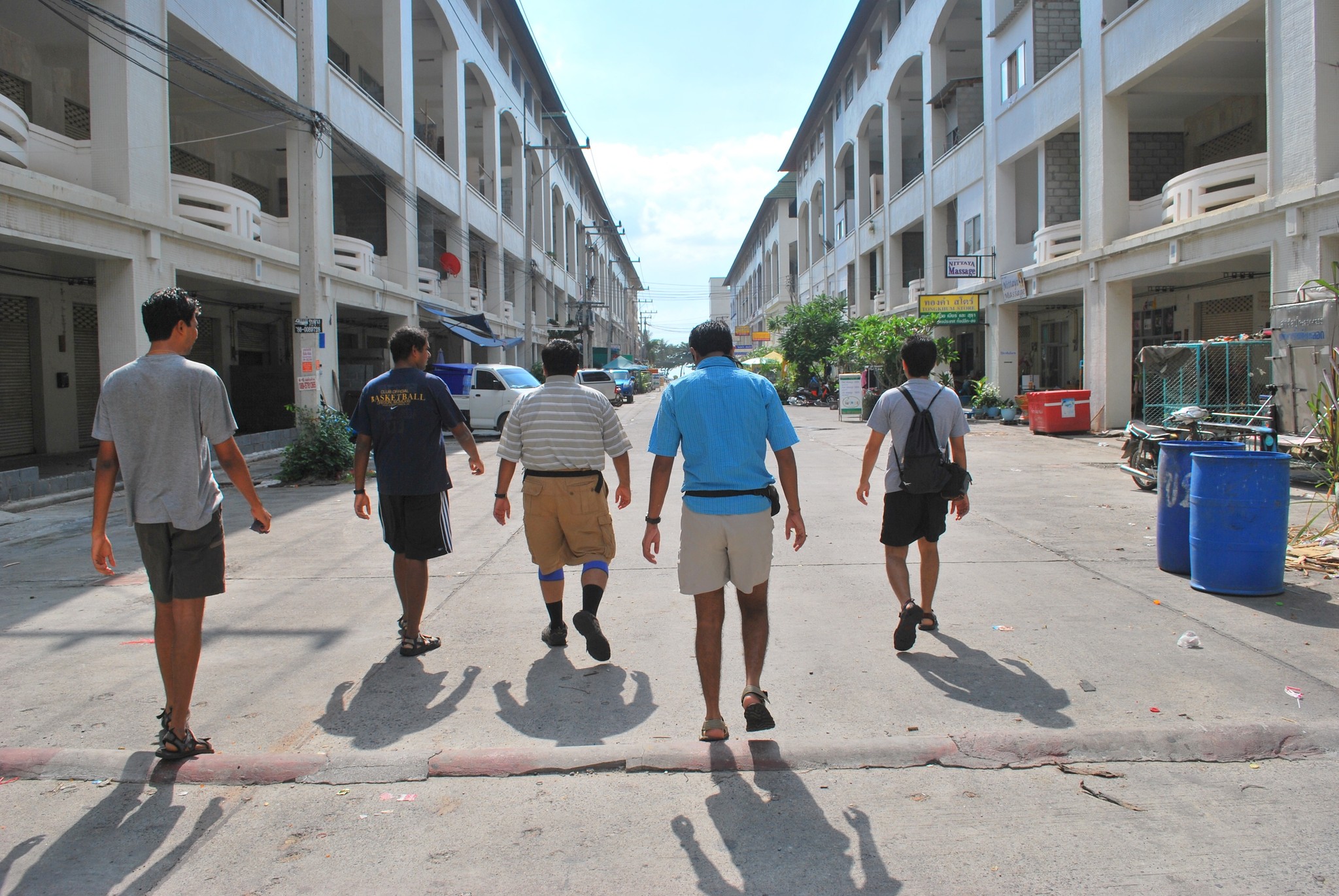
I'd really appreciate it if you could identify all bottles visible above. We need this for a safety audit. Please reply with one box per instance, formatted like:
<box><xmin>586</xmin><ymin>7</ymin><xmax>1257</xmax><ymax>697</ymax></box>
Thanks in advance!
<box><xmin>462</xmin><ymin>375</ymin><xmax>472</xmax><ymax>395</ymax></box>
<box><xmin>1029</xmin><ymin>382</ymin><xmax>1033</xmax><ymax>389</ymax></box>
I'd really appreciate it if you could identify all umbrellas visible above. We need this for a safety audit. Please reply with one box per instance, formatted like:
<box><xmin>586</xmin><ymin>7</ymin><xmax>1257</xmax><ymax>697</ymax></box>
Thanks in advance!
<box><xmin>740</xmin><ymin>357</ymin><xmax>781</xmax><ymax>366</ymax></box>
<box><xmin>434</xmin><ymin>348</ymin><xmax>446</xmax><ymax>370</ymax></box>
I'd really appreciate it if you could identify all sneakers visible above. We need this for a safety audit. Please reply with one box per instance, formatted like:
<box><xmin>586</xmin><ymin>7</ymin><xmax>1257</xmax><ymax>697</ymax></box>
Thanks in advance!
<box><xmin>542</xmin><ymin>620</ymin><xmax>568</xmax><ymax>646</ymax></box>
<box><xmin>573</xmin><ymin>610</ymin><xmax>611</xmax><ymax>661</ymax></box>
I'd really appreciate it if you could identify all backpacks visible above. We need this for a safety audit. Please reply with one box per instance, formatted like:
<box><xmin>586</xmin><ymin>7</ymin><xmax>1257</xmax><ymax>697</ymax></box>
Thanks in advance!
<box><xmin>892</xmin><ymin>385</ymin><xmax>952</xmax><ymax>494</ymax></box>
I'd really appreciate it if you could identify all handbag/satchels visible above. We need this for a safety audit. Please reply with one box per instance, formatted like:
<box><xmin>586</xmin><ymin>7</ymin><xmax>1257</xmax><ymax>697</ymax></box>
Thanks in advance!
<box><xmin>685</xmin><ymin>485</ymin><xmax>781</xmax><ymax>517</ymax></box>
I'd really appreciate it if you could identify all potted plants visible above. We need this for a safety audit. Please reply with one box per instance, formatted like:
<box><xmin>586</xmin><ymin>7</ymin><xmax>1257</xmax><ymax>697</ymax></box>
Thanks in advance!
<box><xmin>969</xmin><ymin>376</ymin><xmax>1018</xmax><ymax>421</ymax></box>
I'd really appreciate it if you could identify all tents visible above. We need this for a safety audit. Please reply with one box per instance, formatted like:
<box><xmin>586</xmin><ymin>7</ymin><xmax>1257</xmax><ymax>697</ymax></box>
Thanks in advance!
<box><xmin>601</xmin><ymin>356</ymin><xmax>648</xmax><ymax>393</ymax></box>
<box><xmin>763</xmin><ymin>351</ymin><xmax>783</xmax><ymax>363</ymax></box>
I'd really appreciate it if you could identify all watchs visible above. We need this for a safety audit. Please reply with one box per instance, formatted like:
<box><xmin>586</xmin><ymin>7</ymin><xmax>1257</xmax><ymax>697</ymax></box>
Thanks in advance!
<box><xmin>353</xmin><ymin>488</ymin><xmax>366</xmax><ymax>494</ymax></box>
<box><xmin>645</xmin><ymin>512</ymin><xmax>662</xmax><ymax>524</ymax></box>
<box><xmin>494</xmin><ymin>491</ymin><xmax>507</xmax><ymax>498</ymax></box>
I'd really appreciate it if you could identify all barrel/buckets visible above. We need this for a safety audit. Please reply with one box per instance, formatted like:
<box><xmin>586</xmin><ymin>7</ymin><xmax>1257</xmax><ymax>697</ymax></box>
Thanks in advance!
<box><xmin>1157</xmin><ymin>440</ymin><xmax>1245</xmax><ymax>574</ymax></box>
<box><xmin>1190</xmin><ymin>450</ymin><xmax>1293</xmax><ymax>595</ymax></box>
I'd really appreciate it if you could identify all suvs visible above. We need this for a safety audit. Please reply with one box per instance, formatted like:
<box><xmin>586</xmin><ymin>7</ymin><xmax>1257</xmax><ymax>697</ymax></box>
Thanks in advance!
<box><xmin>574</xmin><ymin>368</ymin><xmax>617</xmax><ymax>399</ymax></box>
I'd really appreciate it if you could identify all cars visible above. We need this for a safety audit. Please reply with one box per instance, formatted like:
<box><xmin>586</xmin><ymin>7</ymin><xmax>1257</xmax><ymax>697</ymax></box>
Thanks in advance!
<box><xmin>609</xmin><ymin>369</ymin><xmax>635</xmax><ymax>403</ymax></box>
<box><xmin>664</xmin><ymin>375</ymin><xmax>673</xmax><ymax>380</ymax></box>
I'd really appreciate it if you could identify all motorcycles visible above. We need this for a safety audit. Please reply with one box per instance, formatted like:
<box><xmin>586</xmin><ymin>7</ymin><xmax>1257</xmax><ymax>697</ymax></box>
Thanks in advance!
<box><xmin>1120</xmin><ymin>405</ymin><xmax>1217</xmax><ymax>491</ymax></box>
<box><xmin>609</xmin><ymin>389</ymin><xmax>624</xmax><ymax>407</ymax></box>
<box><xmin>634</xmin><ymin>382</ymin><xmax>653</xmax><ymax>393</ymax></box>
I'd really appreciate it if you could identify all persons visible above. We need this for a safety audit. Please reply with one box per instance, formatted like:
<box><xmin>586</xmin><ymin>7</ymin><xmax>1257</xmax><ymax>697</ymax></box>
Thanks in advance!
<box><xmin>969</xmin><ymin>368</ymin><xmax>984</xmax><ymax>381</ymax></box>
<box><xmin>494</xmin><ymin>338</ymin><xmax>633</xmax><ymax>662</ymax></box>
<box><xmin>856</xmin><ymin>340</ymin><xmax>969</xmax><ymax>651</ymax></box>
<box><xmin>90</xmin><ymin>289</ymin><xmax>274</xmax><ymax>760</ymax></box>
<box><xmin>349</xmin><ymin>326</ymin><xmax>485</xmax><ymax>655</ymax></box>
<box><xmin>821</xmin><ymin>383</ymin><xmax>831</xmax><ymax>403</ymax></box>
<box><xmin>641</xmin><ymin>320</ymin><xmax>810</xmax><ymax>742</ymax></box>
<box><xmin>807</xmin><ymin>372</ymin><xmax>822</xmax><ymax>398</ymax></box>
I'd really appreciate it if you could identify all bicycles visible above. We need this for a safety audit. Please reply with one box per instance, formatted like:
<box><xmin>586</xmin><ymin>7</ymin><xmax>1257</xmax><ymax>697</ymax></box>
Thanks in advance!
<box><xmin>787</xmin><ymin>395</ymin><xmax>829</xmax><ymax>407</ymax></box>
<box><xmin>664</xmin><ymin>378</ymin><xmax>669</xmax><ymax>384</ymax></box>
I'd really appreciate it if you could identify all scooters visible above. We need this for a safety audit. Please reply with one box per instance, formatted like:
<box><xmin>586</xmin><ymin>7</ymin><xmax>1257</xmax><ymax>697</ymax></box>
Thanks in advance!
<box><xmin>796</xmin><ymin>375</ymin><xmax>839</xmax><ymax>404</ymax></box>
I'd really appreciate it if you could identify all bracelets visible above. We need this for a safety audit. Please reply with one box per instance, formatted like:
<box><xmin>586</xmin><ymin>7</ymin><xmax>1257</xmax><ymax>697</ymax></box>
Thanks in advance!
<box><xmin>788</xmin><ymin>506</ymin><xmax>801</xmax><ymax>512</ymax></box>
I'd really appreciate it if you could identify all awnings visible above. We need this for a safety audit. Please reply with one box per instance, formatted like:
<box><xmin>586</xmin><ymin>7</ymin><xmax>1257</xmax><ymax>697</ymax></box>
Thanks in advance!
<box><xmin>417</xmin><ymin>303</ymin><xmax>523</xmax><ymax>351</ymax></box>
<box><xmin>685</xmin><ymin>364</ymin><xmax>695</xmax><ymax>367</ymax></box>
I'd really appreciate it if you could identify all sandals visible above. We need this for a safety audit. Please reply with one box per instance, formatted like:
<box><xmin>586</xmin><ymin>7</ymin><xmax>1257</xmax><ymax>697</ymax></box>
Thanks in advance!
<box><xmin>699</xmin><ymin>715</ymin><xmax>729</xmax><ymax>741</ymax></box>
<box><xmin>893</xmin><ymin>598</ymin><xmax>923</xmax><ymax>651</ymax></box>
<box><xmin>156</xmin><ymin>708</ymin><xmax>190</xmax><ymax>729</ymax></box>
<box><xmin>155</xmin><ymin>727</ymin><xmax>215</xmax><ymax>759</ymax></box>
<box><xmin>399</xmin><ymin>631</ymin><xmax>441</xmax><ymax>656</ymax></box>
<box><xmin>918</xmin><ymin>609</ymin><xmax>937</xmax><ymax>630</ymax></box>
<box><xmin>397</xmin><ymin>612</ymin><xmax>407</xmax><ymax>633</ymax></box>
<box><xmin>741</xmin><ymin>686</ymin><xmax>775</xmax><ymax>732</ymax></box>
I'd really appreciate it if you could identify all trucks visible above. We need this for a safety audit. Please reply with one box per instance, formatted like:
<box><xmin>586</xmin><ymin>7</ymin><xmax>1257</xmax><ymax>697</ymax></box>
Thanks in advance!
<box><xmin>452</xmin><ymin>364</ymin><xmax>543</xmax><ymax>434</ymax></box>
